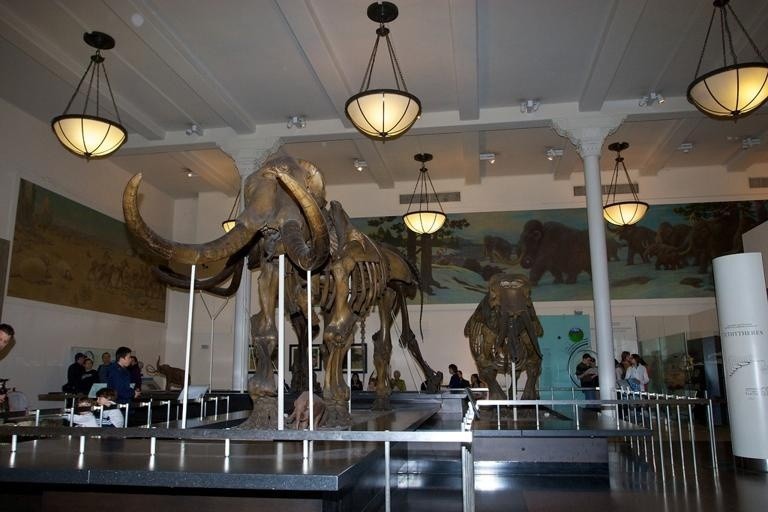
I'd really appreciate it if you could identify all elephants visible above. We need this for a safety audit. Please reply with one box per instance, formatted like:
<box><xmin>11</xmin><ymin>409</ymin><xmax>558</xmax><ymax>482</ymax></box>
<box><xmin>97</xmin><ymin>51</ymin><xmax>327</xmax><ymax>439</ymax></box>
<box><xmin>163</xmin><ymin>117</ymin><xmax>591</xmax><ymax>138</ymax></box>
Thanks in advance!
<box><xmin>145</xmin><ymin>356</ymin><xmax>191</xmax><ymax>392</ymax></box>
<box><xmin>492</xmin><ymin>219</ymin><xmax>592</xmax><ymax>287</ymax></box>
<box><xmin>483</xmin><ymin>236</ymin><xmax>510</xmax><ymax>263</ymax></box>
<box><xmin>462</xmin><ymin>272</ymin><xmax>544</xmax><ymax>409</ymax></box>
<box><xmin>607</xmin><ymin>215</ymin><xmax>759</xmax><ymax>274</ymax></box>
<box><xmin>122</xmin><ymin>156</ymin><xmax>332</xmax><ymax>273</ymax></box>
<box><xmin>287</xmin><ymin>391</ymin><xmax>325</xmax><ymax>430</ymax></box>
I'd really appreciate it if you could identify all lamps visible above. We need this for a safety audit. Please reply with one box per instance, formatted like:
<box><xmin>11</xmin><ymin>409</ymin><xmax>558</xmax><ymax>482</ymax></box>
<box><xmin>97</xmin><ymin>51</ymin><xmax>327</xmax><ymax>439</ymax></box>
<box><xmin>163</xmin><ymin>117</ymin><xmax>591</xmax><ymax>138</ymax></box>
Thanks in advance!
<box><xmin>402</xmin><ymin>152</ymin><xmax>449</xmax><ymax>236</ymax></box>
<box><xmin>685</xmin><ymin>62</ymin><xmax>768</xmax><ymax>122</ymax></box>
<box><xmin>600</xmin><ymin>140</ymin><xmax>650</xmax><ymax>227</ymax></box>
<box><xmin>342</xmin><ymin>2</ymin><xmax>424</xmax><ymax>145</ymax></box>
<box><xmin>50</xmin><ymin>27</ymin><xmax>129</xmax><ymax>164</ymax></box>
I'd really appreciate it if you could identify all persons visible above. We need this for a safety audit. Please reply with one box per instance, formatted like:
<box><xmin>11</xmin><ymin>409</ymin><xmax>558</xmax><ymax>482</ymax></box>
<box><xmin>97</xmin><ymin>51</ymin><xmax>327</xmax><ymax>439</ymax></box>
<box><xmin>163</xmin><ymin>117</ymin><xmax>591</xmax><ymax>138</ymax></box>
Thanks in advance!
<box><xmin>457</xmin><ymin>371</ymin><xmax>470</xmax><ymax>388</ymax></box>
<box><xmin>67</xmin><ymin>347</ymin><xmax>142</xmax><ymax>428</ymax></box>
<box><xmin>470</xmin><ymin>374</ymin><xmax>485</xmax><ymax>388</ymax></box>
<box><xmin>421</xmin><ymin>374</ymin><xmax>440</xmax><ymax>392</ymax></box>
<box><xmin>390</xmin><ymin>370</ymin><xmax>406</xmax><ymax>392</ymax></box>
<box><xmin>448</xmin><ymin>364</ymin><xmax>460</xmax><ymax>394</ymax></box>
<box><xmin>0</xmin><ymin>323</ymin><xmax>14</xmax><ymax>354</ymax></box>
<box><xmin>575</xmin><ymin>351</ymin><xmax>650</xmax><ymax>422</ymax></box>
<box><xmin>351</xmin><ymin>373</ymin><xmax>363</xmax><ymax>391</ymax></box>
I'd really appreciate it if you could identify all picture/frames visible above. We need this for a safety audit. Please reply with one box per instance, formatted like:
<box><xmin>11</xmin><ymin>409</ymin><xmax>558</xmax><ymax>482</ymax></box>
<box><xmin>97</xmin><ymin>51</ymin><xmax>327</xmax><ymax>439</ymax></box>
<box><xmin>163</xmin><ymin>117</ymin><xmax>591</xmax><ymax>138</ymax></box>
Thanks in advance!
<box><xmin>341</xmin><ymin>342</ymin><xmax>369</xmax><ymax>372</ymax></box>
<box><xmin>247</xmin><ymin>343</ymin><xmax>280</xmax><ymax>375</ymax></box>
<box><xmin>288</xmin><ymin>343</ymin><xmax>323</xmax><ymax>373</ymax></box>
